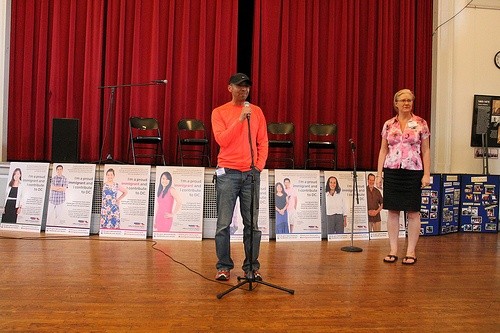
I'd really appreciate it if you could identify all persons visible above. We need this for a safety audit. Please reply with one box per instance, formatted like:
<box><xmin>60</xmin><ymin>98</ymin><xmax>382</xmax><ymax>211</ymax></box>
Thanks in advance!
<box><xmin>47</xmin><ymin>165</ymin><xmax>69</xmax><ymax>224</ymax></box>
<box><xmin>211</xmin><ymin>74</ymin><xmax>269</xmax><ymax>281</ymax></box>
<box><xmin>366</xmin><ymin>173</ymin><xmax>383</xmax><ymax>231</ymax></box>
<box><xmin>1</xmin><ymin>168</ymin><xmax>25</xmax><ymax>223</ymax></box>
<box><xmin>276</xmin><ymin>178</ymin><xmax>298</xmax><ymax>234</ymax></box>
<box><xmin>376</xmin><ymin>89</ymin><xmax>431</xmax><ymax>265</ymax></box>
<box><xmin>326</xmin><ymin>176</ymin><xmax>347</xmax><ymax>234</ymax></box>
<box><xmin>100</xmin><ymin>169</ymin><xmax>128</xmax><ymax>228</ymax></box>
<box><xmin>155</xmin><ymin>172</ymin><xmax>181</xmax><ymax>231</ymax></box>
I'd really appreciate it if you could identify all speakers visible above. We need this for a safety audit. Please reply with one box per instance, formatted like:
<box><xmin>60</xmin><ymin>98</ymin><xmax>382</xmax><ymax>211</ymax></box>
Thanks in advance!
<box><xmin>51</xmin><ymin>118</ymin><xmax>78</xmax><ymax>162</ymax></box>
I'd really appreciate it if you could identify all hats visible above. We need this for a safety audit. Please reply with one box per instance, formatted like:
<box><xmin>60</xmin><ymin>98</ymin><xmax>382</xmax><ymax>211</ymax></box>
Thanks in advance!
<box><xmin>229</xmin><ymin>74</ymin><xmax>251</xmax><ymax>85</ymax></box>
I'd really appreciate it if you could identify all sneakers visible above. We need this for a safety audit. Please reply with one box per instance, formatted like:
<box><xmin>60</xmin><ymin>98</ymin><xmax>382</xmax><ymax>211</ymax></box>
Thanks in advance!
<box><xmin>214</xmin><ymin>268</ymin><xmax>230</xmax><ymax>280</ymax></box>
<box><xmin>245</xmin><ymin>270</ymin><xmax>262</xmax><ymax>281</ymax></box>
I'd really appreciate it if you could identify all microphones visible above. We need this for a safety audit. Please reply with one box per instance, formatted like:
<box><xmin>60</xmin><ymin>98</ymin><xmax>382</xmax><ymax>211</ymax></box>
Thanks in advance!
<box><xmin>348</xmin><ymin>136</ymin><xmax>357</xmax><ymax>149</ymax></box>
<box><xmin>244</xmin><ymin>101</ymin><xmax>250</xmax><ymax>119</ymax></box>
<box><xmin>152</xmin><ymin>79</ymin><xmax>168</xmax><ymax>84</ymax></box>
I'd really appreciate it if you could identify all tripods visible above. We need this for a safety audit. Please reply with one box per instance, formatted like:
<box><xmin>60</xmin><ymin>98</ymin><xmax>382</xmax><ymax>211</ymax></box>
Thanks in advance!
<box><xmin>216</xmin><ymin>114</ymin><xmax>296</xmax><ymax>298</ymax></box>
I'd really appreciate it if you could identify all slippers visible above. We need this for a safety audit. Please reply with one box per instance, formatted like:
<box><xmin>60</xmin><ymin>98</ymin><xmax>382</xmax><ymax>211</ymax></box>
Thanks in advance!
<box><xmin>384</xmin><ymin>255</ymin><xmax>398</xmax><ymax>262</ymax></box>
<box><xmin>402</xmin><ymin>257</ymin><xmax>417</xmax><ymax>264</ymax></box>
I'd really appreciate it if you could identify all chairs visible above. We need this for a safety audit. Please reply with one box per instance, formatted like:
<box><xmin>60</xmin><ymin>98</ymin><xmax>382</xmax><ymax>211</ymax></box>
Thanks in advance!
<box><xmin>128</xmin><ymin>117</ymin><xmax>166</xmax><ymax>166</ymax></box>
<box><xmin>176</xmin><ymin>119</ymin><xmax>211</xmax><ymax>168</ymax></box>
<box><xmin>267</xmin><ymin>123</ymin><xmax>295</xmax><ymax>170</ymax></box>
<box><xmin>306</xmin><ymin>124</ymin><xmax>338</xmax><ymax>170</ymax></box>
